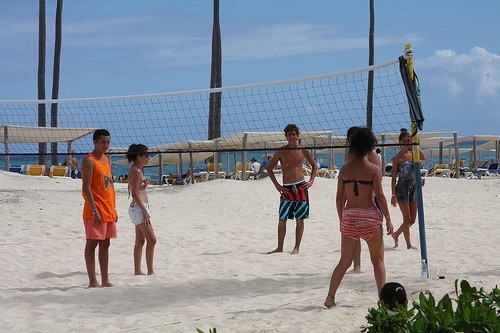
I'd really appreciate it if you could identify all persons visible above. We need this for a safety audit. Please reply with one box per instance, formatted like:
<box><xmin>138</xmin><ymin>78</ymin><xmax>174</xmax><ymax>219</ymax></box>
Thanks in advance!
<box><xmin>391</xmin><ymin>128</ymin><xmax>426</xmax><ymax>250</ymax></box>
<box><xmin>70</xmin><ymin>154</ymin><xmax>81</xmax><ymax>180</ymax></box>
<box><xmin>127</xmin><ymin>144</ymin><xmax>156</xmax><ymax>274</ymax></box>
<box><xmin>379</xmin><ymin>281</ymin><xmax>408</xmax><ymax>311</ymax></box>
<box><xmin>266</xmin><ymin>123</ymin><xmax>316</xmax><ymax>255</ymax></box>
<box><xmin>324</xmin><ymin>126</ymin><xmax>394</xmax><ymax>307</ymax></box>
<box><xmin>81</xmin><ymin>129</ymin><xmax>118</xmax><ymax>288</ymax></box>
<box><xmin>186</xmin><ymin>163</ymin><xmax>193</xmax><ymax>177</ymax></box>
<box><xmin>260</xmin><ymin>156</ymin><xmax>273</xmax><ymax>172</ymax></box>
<box><xmin>248</xmin><ymin>157</ymin><xmax>261</xmax><ymax>174</ymax></box>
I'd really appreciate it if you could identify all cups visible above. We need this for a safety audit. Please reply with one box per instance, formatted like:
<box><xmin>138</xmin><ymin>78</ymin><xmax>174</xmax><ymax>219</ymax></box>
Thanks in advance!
<box><xmin>430</xmin><ymin>268</ymin><xmax>437</xmax><ymax>279</ymax></box>
<box><xmin>439</xmin><ymin>267</ymin><xmax>446</xmax><ymax>279</ymax></box>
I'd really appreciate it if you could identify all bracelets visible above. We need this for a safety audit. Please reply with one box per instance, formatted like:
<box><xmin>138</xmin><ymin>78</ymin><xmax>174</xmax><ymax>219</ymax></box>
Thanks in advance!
<box><xmin>392</xmin><ymin>194</ymin><xmax>395</xmax><ymax>196</ymax></box>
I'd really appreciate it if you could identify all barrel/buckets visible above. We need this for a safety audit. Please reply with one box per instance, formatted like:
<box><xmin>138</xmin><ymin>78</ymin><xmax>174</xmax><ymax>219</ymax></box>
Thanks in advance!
<box><xmin>420</xmin><ymin>259</ymin><xmax>428</xmax><ymax>278</ymax></box>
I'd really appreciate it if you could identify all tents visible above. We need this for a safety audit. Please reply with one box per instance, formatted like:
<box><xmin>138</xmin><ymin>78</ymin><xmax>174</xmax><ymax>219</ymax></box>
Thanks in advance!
<box><xmin>0</xmin><ymin>124</ymin><xmax>103</xmax><ymax>173</ymax></box>
<box><xmin>108</xmin><ymin>130</ymin><xmax>500</xmax><ymax>186</ymax></box>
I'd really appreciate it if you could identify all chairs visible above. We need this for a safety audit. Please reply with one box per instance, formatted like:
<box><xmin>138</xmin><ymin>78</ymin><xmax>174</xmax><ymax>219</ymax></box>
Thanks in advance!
<box><xmin>9</xmin><ymin>165</ymin><xmax>24</xmax><ymax>174</ymax></box>
<box><xmin>46</xmin><ymin>165</ymin><xmax>68</xmax><ymax>179</ymax></box>
<box><xmin>161</xmin><ymin>169</ymin><xmax>226</xmax><ymax>186</ymax></box>
<box><xmin>71</xmin><ymin>168</ymin><xmax>82</xmax><ymax>179</ymax></box>
<box><xmin>302</xmin><ymin>162</ymin><xmax>339</xmax><ymax>179</ymax></box>
<box><xmin>120</xmin><ymin>176</ymin><xmax>128</xmax><ymax>184</ymax></box>
<box><xmin>231</xmin><ymin>161</ymin><xmax>282</xmax><ymax>181</ymax></box>
<box><xmin>25</xmin><ymin>164</ymin><xmax>45</xmax><ymax>176</ymax></box>
<box><xmin>420</xmin><ymin>159</ymin><xmax>500</xmax><ymax>179</ymax></box>
